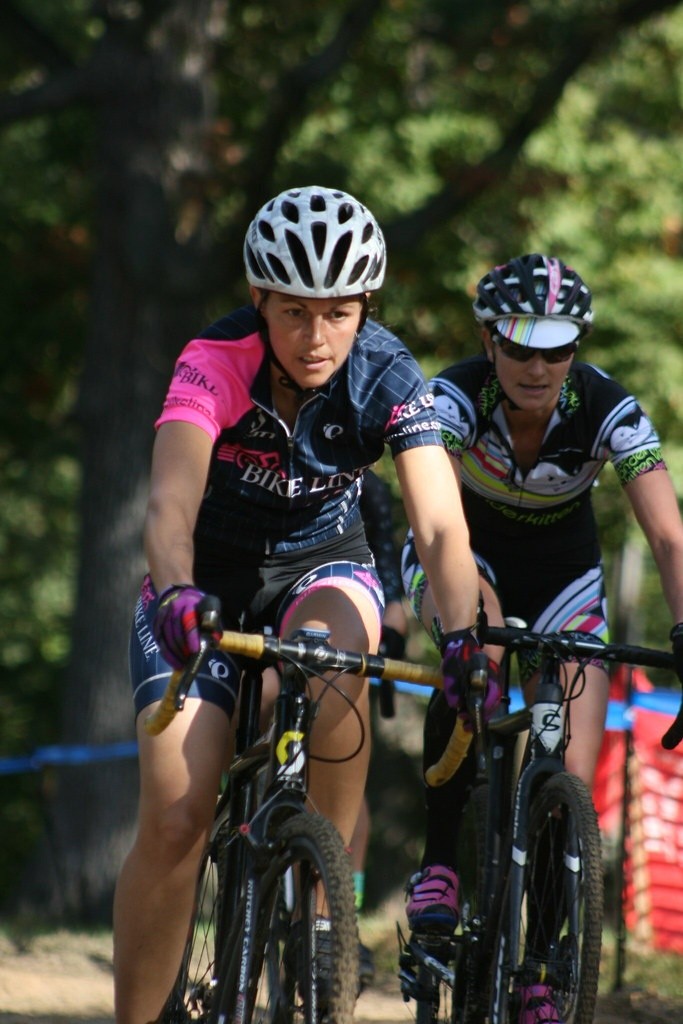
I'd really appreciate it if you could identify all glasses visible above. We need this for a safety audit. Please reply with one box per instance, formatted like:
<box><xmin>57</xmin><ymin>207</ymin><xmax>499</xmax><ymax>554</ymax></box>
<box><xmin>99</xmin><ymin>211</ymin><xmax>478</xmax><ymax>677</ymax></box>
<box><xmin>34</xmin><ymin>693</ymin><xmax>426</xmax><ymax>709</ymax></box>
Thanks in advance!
<box><xmin>491</xmin><ymin>331</ymin><xmax>581</xmax><ymax>363</ymax></box>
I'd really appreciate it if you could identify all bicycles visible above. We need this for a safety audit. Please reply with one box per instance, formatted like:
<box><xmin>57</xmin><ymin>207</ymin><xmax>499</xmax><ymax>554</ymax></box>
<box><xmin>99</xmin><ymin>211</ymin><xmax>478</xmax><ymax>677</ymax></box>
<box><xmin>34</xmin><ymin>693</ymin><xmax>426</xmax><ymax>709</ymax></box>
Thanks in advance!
<box><xmin>142</xmin><ymin>594</ymin><xmax>496</xmax><ymax>1024</ymax></box>
<box><xmin>413</xmin><ymin>607</ymin><xmax>680</xmax><ymax>1024</ymax></box>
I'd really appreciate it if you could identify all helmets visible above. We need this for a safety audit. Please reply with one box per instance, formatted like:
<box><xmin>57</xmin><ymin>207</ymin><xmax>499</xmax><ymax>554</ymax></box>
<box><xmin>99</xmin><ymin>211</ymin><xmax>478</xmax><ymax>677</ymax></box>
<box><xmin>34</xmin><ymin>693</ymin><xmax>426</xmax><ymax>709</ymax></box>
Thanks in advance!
<box><xmin>241</xmin><ymin>185</ymin><xmax>388</xmax><ymax>299</ymax></box>
<box><xmin>472</xmin><ymin>254</ymin><xmax>595</xmax><ymax>328</ymax></box>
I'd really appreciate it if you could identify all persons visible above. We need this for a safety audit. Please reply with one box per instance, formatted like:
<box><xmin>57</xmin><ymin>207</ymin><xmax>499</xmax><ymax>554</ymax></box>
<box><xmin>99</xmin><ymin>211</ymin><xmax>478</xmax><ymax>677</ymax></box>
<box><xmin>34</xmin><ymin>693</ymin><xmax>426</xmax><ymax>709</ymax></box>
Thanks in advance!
<box><xmin>215</xmin><ymin>468</ymin><xmax>410</xmax><ymax>918</ymax></box>
<box><xmin>105</xmin><ymin>182</ymin><xmax>502</xmax><ymax>1024</ymax></box>
<box><xmin>398</xmin><ymin>253</ymin><xmax>683</xmax><ymax>1024</ymax></box>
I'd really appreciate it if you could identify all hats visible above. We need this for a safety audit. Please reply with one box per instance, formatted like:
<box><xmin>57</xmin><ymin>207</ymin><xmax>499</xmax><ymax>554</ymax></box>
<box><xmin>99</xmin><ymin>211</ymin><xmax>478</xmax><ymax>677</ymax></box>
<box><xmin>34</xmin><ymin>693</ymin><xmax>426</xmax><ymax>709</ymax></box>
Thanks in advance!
<box><xmin>492</xmin><ymin>274</ymin><xmax>582</xmax><ymax>349</ymax></box>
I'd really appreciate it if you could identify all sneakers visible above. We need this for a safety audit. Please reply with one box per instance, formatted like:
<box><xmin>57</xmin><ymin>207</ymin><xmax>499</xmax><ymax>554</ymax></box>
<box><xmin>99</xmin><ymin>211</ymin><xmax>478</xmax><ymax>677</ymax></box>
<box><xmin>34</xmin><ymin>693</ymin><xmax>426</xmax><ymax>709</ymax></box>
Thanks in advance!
<box><xmin>510</xmin><ymin>977</ymin><xmax>565</xmax><ymax>1024</ymax></box>
<box><xmin>403</xmin><ymin>864</ymin><xmax>462</xmax><ymax>933</ymax></box>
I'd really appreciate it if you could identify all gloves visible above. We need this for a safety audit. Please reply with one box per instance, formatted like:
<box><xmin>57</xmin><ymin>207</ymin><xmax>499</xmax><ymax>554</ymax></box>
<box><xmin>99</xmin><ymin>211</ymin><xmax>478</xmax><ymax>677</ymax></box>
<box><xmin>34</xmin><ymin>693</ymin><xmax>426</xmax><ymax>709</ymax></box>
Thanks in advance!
<box><xmin>440</xmin><ymin>632</ymin><xmax>505</xmax><ymax>734</ymax></box>
<box><xmin>669</xmin><ymin>622</ymin><xmax>683</xmax><ymax>686</ymax></box>
<box><xmin>151</xmin><ymin>583</ymin><xmax>224</xmax><ymax>671</ymax></box>
